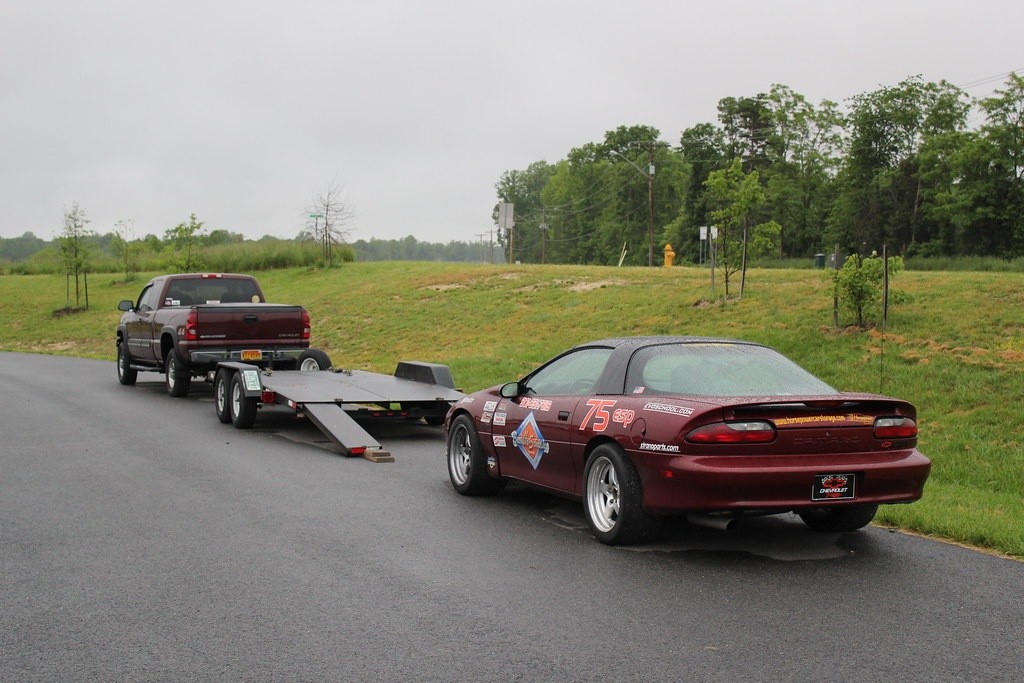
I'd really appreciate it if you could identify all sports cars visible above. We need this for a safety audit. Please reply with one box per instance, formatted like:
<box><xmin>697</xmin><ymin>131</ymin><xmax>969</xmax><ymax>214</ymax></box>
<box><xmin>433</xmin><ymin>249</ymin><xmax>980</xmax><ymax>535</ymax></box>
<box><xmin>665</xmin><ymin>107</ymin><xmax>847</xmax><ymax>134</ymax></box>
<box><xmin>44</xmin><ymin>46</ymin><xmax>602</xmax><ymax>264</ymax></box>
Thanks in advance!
<box><xmin>443</xmin><ymin>333</ymin><xmax>932</xmax><ymax>548</ymax></box>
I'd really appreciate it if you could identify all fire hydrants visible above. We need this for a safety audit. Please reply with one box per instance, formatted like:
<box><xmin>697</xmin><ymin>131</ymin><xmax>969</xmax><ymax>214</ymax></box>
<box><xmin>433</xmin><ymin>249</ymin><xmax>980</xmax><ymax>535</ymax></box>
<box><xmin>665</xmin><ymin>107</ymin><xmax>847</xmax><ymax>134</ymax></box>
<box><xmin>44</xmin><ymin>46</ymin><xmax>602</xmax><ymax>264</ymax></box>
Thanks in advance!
<box><xmin>662</xmin><ymin>243</ymin><xmax>675</xmax><ymax>269</ymax></box>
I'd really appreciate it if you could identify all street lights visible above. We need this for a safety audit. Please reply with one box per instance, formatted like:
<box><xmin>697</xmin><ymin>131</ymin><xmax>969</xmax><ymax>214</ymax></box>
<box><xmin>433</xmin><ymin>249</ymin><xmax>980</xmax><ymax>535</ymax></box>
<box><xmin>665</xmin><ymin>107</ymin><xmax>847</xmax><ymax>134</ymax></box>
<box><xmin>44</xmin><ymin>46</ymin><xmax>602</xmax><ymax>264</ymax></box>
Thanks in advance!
<box><xmin>609</xmin><ymin>150</ymin><xmax>655</xmax><ymax>267</ymax></box>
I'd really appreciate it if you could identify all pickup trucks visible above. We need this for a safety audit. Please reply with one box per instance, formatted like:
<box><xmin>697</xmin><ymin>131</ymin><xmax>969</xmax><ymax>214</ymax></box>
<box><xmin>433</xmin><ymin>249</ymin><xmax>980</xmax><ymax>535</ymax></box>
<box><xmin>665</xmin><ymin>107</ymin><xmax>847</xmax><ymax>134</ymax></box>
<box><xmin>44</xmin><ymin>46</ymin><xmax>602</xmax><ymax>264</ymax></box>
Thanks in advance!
<box><xmin>114</xmin><ymin>273</ymin><xmax>310</xmax><ymax>399</ymax></box>
